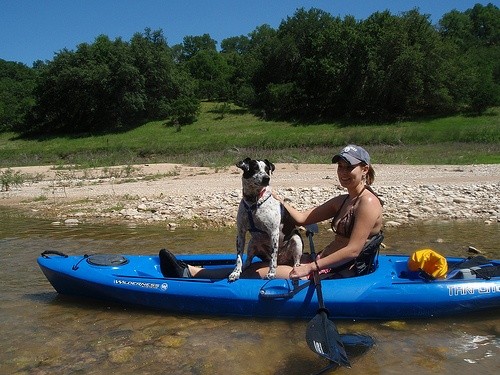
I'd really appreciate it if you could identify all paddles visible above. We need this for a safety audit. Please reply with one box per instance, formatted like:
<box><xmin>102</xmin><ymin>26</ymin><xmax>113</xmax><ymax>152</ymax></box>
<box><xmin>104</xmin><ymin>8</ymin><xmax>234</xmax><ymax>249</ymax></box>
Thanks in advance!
<box><xmin>295</xmin><ymin>220</ymin><xmax>352</xmax><ymax>369</ymax></box>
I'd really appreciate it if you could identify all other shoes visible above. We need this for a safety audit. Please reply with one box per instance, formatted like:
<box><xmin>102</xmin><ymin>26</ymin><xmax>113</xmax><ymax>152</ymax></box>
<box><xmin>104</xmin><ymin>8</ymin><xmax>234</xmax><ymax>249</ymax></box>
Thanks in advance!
<box><xmin>159</xmin><ymin>249</ymin><xmax>192</xmax><ymax>278</ymax></box>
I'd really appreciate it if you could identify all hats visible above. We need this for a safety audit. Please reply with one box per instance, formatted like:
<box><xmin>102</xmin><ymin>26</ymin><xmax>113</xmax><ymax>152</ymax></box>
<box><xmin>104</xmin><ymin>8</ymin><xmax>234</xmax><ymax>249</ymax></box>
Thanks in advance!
<box><xmin>332</xmin><ymin>145</ymin><xmax>370</xmax><ymax>167</ymax></box>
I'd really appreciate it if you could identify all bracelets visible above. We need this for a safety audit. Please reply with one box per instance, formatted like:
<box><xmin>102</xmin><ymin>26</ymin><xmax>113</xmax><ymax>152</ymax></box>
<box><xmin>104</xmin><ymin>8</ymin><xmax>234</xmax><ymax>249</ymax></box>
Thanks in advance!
<box><xmin>315</xmin><ymin>260</ymin><xmax>321</xmax><ymax>271</ymax></box>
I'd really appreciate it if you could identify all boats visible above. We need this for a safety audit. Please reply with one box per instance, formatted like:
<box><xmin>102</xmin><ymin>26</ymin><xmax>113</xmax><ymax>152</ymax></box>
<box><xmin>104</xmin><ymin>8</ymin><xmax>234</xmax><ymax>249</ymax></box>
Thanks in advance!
<box><xmin>37</xmin><ymin>250</ymin><xmax>500</xmax><ymax>321</ymax></box>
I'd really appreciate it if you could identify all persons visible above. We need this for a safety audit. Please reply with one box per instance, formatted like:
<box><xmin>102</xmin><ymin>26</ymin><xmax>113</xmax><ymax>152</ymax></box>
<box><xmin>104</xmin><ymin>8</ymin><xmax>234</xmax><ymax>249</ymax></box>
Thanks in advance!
<box><xmin>159</xmin><ymin>145</ymin><xmax>382</xmax><ymax>280</ymax></box>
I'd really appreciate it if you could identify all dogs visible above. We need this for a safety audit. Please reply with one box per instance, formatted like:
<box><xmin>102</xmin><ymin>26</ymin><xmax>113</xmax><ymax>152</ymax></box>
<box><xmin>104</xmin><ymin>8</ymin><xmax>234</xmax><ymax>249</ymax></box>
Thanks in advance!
<box><xmin>228</xmin><ymin>157</ymin><xmax>304</xmax><ymax>284</ymax></box>
